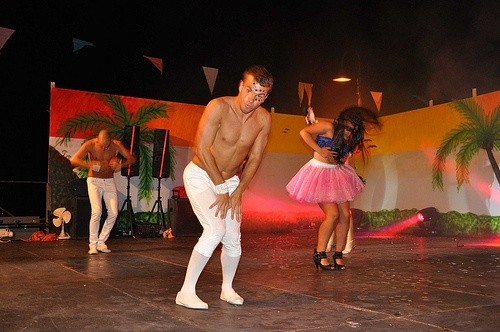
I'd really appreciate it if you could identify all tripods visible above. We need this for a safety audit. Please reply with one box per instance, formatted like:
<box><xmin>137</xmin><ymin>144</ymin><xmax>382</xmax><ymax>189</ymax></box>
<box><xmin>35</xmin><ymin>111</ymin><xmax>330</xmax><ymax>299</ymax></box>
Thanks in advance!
<box><xmin>112</xmin><ymin>177</ymin><xmax>138</xmax><ymax>238</ymax></box>
<box><xmin>142</xmin><ymin>178</ymin><xmax>168</xmax><ymax>239</ymax></box>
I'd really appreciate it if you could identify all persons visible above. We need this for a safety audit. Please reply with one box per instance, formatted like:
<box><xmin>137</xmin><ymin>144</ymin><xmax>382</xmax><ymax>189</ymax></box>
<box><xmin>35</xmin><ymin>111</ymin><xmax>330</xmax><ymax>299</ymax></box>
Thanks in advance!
<box><xmin>175</xmin><ymin>65</ymin><xmax>273</xmax><ymax>309</ymax></box>
<box><xmin>285</xmin><ymin>105</ymin><xmax>383</xmax><ymax>270</ymax></box>
<box><xmin>69</xmin><ymin>129</ymin><xmax>135</xmax><ymax>255</ymax></box>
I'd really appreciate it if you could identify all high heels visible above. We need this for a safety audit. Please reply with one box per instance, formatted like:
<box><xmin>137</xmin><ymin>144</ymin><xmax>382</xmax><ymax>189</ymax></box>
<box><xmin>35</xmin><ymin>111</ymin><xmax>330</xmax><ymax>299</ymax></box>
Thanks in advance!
<box><xmin>333</xmin><ymin>252</ymin><xmax>346</xmax><ymax>270</ymax></box>
<box><xmin>325</xmin><ymin>243</ymin><xmax>336</xmax><ymax>253</ymax></box>
<box><xmin>342</xmin><ymin>238</ymin><xmax>358</xmax><ymax>255</ymax></box>
<box><xmin>313</xmin><ymin>248</ymin><xmax>335</xmax><ymax>270</ymax></box>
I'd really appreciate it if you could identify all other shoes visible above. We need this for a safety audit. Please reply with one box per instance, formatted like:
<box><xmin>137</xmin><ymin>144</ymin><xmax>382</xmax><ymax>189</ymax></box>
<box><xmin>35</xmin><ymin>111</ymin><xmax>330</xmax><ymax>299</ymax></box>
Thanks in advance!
<box><xmin>89</xmin><ymin>245</ymin><xmax>99</xmax><ymax>254</ymax></box>
<box><xmin>175</xmin><ymin>292</ymin><xmax>208</xmax><ymax>309</ymax></box>
<box><xmin>220</xmin><ymin>292</ymin><xmax>244</xmax><ymax>305</ymax></box>
<box><xmin>97</xmin><ymin>243</ymin><xmax>111</xmax><ymax>252</ymax></box>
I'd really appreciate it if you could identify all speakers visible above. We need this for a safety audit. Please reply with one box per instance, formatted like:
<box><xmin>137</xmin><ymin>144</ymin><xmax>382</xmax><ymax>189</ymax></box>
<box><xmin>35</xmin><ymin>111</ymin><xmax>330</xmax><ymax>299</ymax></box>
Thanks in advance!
<box><xmin>168</xmin><ymin>198</ymin><xmax>204</xmax><ymax>236</ymax></box>
<box><xmin>67</xmin><ymin>195</ymin><xmax>106</xmax><ymax>239</ymax></box>
<box><xmin>121</xmin><ymin>125</ymin><xmax>140</xmax><ymax>177</ymax></box>
<box><xmin>152</xmin><ymin>129</ymin><xmax>171</xmax><ymax>178</ymax></box>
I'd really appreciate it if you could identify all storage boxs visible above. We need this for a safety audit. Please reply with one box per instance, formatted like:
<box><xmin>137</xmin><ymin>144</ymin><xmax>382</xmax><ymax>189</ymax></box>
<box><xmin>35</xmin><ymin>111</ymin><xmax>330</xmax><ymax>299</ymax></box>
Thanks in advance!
<box><xmin>136</xmin><ymin>222</ymin><xmax>161</xmax><ymax>237</ymax></box>
<box><xmin>70</xmin><ymin>196</ymin><xmax>107</xmax><ymax>240</ymax></box>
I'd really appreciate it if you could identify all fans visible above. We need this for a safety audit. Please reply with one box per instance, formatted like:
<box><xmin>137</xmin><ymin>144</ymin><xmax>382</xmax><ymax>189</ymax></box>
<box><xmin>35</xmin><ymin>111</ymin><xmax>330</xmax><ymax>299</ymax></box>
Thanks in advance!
<box><xmin>52</xmin><ymin>207</ymin><xmax>72</xmax><ymax>239</ymax></box>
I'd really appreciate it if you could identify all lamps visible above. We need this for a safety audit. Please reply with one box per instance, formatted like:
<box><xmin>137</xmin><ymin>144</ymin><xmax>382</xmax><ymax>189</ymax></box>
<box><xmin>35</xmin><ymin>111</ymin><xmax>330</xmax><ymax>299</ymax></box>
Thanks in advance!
<box><xmin>415</xmin><ymin>207</ymin><xmax>440</xmax><ymax>236</ymax></box>
<box><xmin>350</xmin><ymin>207</ymin><xmax>370</xmax><ymax>229</ymax></box>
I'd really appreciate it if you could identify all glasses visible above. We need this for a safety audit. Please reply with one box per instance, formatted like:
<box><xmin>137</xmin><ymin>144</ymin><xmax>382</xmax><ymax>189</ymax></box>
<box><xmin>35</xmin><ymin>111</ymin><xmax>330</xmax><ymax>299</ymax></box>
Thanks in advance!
<box><xmin>345</xmin><ymin>125</ymin><xmax>356</xmax><ymax>134</ymax></box>
